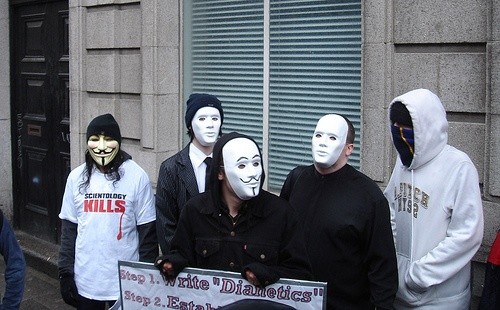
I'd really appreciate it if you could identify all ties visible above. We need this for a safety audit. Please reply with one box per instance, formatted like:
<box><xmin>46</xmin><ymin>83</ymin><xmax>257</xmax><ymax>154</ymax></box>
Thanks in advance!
<box><xmin>203</xmin><ymin>157</ymin><xmax>212</xmax><ymax>191</ymax></box>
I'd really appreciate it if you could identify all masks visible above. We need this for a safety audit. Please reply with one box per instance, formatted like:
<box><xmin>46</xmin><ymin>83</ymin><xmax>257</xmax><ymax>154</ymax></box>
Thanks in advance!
<box><xmin>391</xmin><ymin>124</ymin><xmax>414</xmax><ymax>165</ymax></box>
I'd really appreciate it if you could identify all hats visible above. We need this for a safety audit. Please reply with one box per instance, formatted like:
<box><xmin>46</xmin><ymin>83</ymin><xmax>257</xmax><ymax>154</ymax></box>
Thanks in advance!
<box><xmin>185</xmin><ymin>93</ymin><xmax>223</xmax><ymax>127</ymax></box>
<box><xmin>86</xmin><ymin>113</ymin><xmax>122</xmax><ymax>144</ymax></box>
<box><xmin>390</xmin><ymin>102</ymin><xmax>413</xmax><ymax>126</ymax></box>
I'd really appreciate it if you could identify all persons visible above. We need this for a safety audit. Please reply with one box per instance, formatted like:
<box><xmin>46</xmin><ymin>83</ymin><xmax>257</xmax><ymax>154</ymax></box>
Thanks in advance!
<box><xmin>383</xmin><ymin>87</ymin><xmax>484</xmax><ymax>310</ymax></box>
<box><xmin>57</xmin><ymin>113</ymin><xmax>156</xmax><ymax>310</ymax></box>
<box><xmin>155</xmin><ymin>93</ymin><xmax>232</xmax><ymax>256</ymax></box>
<box><xmin>279</xmin><ymin>113</ymin><xmax>398</xmax><ymax>310</ymax></box>
<box><xmin>0</xmin><ymin>208</ymin><xmax>26</xmax><ymax>310</ymax></box>
<box><xmin>154</xmin><ymin>131</ymin><xmax>286</xmax><ymax>286</ymax></box>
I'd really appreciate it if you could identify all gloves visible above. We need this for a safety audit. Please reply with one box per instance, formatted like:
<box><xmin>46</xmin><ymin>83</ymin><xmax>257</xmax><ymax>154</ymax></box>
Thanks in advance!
<box><xmin>59</xmin><ymin>272</ymin><xmax>79</xmax><ymax>308</ymax></box>
